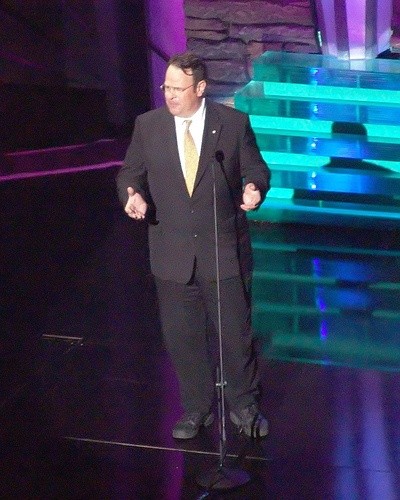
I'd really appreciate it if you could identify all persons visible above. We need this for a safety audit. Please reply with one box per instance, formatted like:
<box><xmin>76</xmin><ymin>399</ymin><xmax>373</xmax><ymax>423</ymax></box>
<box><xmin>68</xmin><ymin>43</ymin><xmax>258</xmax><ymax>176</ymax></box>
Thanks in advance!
<box><xmin>117</xmin><ymin>53</ymin><xmax>273</xmax><ymax>439</ymax></box>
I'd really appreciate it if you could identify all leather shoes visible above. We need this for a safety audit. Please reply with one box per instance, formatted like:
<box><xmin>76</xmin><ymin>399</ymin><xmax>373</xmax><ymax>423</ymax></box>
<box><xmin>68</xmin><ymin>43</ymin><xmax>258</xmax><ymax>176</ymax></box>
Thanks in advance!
<box><xmin>170</xmin><ymin>409</ymin><xmax>215</xmax><ymax>442</ymax></box>
<box><xmin>230</xmin><ymin>407</ymin><xmax>269</xmax><ymax>439</ymax></box>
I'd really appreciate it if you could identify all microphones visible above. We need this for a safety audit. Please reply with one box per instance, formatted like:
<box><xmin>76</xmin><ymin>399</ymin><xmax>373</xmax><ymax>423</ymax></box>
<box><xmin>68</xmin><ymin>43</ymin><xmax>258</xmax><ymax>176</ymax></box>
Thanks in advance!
<box><xmin>206</xmin><ymin>157</ymin><xmax>219</xmax><ymax>189</ymax></box>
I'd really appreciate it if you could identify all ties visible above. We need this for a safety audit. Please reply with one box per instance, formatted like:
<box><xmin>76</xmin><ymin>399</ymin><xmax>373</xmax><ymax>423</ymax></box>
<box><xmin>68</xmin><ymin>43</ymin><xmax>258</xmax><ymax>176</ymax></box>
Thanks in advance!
<box><xmin>182</xmin><ymin>118</ymin><xmax>199</xmax><ymax>198</ymax></box>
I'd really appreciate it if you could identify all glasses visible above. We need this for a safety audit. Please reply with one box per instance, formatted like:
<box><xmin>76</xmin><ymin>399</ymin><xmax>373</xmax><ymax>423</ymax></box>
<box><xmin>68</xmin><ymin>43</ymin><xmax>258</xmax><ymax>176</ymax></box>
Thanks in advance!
<box><xmin>158</xmin><ymin>83</ymin><xmax>195</xmax><ymax>94</ymax></box>
